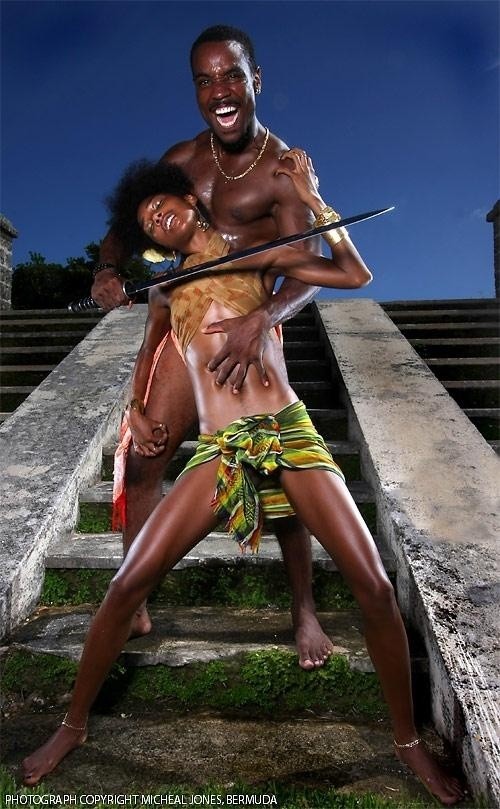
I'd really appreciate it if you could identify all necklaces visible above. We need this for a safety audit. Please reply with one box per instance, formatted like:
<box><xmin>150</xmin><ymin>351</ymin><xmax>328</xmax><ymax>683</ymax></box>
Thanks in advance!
<box><xmin>205</xmin><ymin>127</ymin><xmax>271</xmax><ymax>182</ymax></box>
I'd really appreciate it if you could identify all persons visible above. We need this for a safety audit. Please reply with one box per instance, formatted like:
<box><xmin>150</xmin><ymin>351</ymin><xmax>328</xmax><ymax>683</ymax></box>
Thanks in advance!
<box><xmin>88</xmin><ymin>22</ymin><xmax>336</xmax><ymax>669</ymax></box>
<box><xmin>17</xmin><ymin>146</ymin><xmax>467</xmax><ymax>802</ymax></box>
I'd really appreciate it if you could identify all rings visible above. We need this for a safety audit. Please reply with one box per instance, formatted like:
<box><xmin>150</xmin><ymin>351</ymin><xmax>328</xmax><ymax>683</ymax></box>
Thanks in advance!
<box><xmin>154</xmin><ymin>440</ymin><xmax>162</xmax><ymax>447</ymax></box>
<box><xmin>160</xmin><ymin>423</ymin><xmax>164</xmax><ymax>429</ymax></box>
<box><xmin>152</xmin><ymin>446</ymin><xmax>156</xmax><ymax>451</ymax></box>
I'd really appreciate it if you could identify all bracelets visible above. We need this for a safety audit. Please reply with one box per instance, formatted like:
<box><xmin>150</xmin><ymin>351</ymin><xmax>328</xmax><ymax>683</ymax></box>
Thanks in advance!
<box><xmin>122</xmin><ymin>398</ymin><xmax>143</xmax><ymax>418</ymax></box>
<box><xmin>306</xmin><ymin>204</ymin><xmax>348</xmax><ymax>254</ymax></box>
<box><xmin>89</xmin><ymin>260</ymin><xmax>119</xmax><ymax>273</ymax></box>
